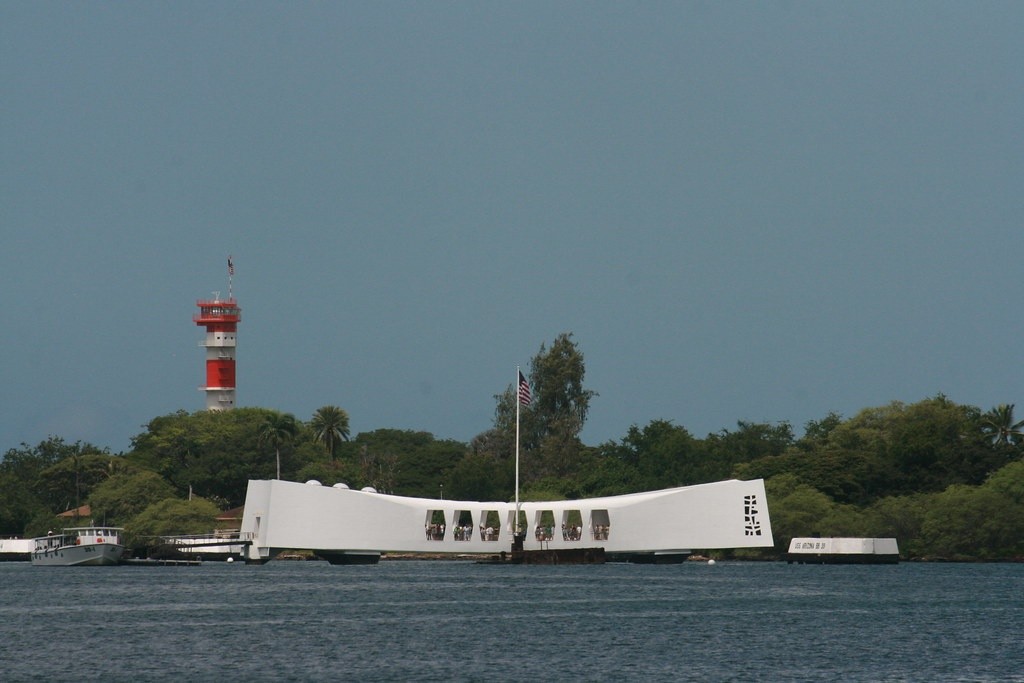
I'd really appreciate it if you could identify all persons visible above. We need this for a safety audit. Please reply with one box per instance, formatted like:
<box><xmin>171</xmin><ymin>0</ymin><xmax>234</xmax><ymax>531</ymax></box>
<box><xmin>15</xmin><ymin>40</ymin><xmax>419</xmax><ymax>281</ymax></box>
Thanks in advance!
<box><xmin>594</xmin><ymin>524</ymin><xmax>609</xmax><ymax>540</ymax></box>
<box><xmin>453</xmin><ymin>524</ymin><xmax>473</xmax><ymax>541</ymax></box>
<box><xmin>513</xmin><ymin>523</ymin><xmax>526</xmax><ymax>541</ymax></box>
<box><xmin>562</xmin><ymin>521</ymin><xmax>581</xmax><ymax>541</ymax></box>
<box><xmin>425</xmin><ymin>521</ymin><xmax>445</xmax><ymax>540</ymax></box>
<box><xmin>480</xmin><ymin>522</ymin><xmax>499</xmax><ymax>541</ymax></box>
<box><xmin>535</xmin><ymin>524</ymin><xmax>555</xmax><ymax>540</ymax></box>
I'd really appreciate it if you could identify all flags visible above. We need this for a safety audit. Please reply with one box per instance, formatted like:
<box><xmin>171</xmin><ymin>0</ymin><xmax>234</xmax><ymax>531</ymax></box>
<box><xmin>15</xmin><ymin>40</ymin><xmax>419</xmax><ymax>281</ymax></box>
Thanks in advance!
<box><xmin>519</xmin><ymin>371</ymin><xmax>530</xmax><ymax>404</ymax></box>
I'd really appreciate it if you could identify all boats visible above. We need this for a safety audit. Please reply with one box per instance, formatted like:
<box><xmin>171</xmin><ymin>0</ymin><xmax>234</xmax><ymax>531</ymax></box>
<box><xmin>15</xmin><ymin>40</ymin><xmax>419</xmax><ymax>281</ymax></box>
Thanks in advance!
<box><xmin>30</xmin><ymin>526</ymin><xmax>127</xmax><ymax>566</ymax></box>
<box><xmin>686</xmin><ymin>555</ymin><xmax>710</xmax><ymax>561</ymax></box>
